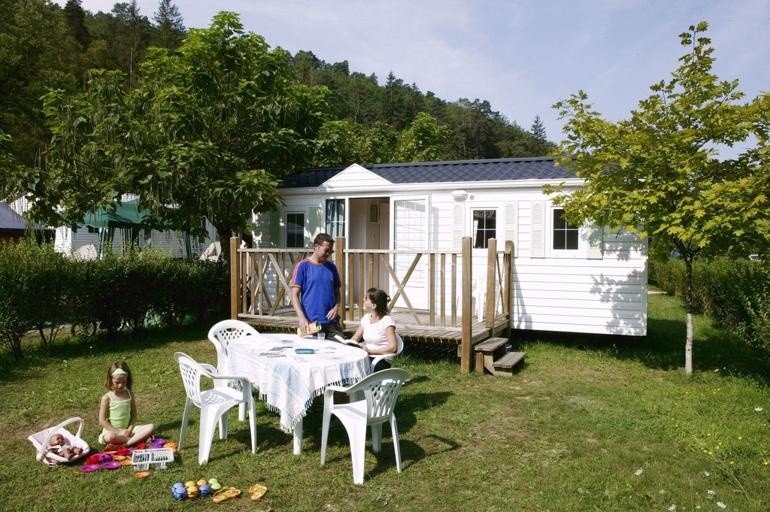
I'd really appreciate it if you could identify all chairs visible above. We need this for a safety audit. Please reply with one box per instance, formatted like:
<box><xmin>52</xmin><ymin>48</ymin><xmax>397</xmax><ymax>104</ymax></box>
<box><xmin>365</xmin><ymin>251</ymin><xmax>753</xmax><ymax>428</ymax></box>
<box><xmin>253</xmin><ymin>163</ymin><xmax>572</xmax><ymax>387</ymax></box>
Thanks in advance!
<box><xmin>173</xmin><ymin>318</ymin><xmax>408</xmax><ymax>485</ymax></box>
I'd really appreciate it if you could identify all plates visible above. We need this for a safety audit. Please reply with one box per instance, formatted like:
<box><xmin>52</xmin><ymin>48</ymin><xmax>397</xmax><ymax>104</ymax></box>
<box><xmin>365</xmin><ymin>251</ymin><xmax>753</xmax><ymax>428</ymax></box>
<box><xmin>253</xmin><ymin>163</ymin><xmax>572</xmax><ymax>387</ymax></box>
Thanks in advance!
<box><xmin>80</xmin><ymin>438</ymin><xmax>176</xmax><ymax>478</ymax></box>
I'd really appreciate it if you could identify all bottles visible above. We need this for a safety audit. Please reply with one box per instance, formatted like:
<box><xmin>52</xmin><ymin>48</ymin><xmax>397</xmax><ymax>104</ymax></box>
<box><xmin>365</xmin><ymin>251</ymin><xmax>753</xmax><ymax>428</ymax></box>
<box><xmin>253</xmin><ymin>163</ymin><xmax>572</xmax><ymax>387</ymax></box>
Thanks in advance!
<box><xmin>297</xmin><ymin>321</ymin><xmax>322</xmax><ymax>337</ymax></box>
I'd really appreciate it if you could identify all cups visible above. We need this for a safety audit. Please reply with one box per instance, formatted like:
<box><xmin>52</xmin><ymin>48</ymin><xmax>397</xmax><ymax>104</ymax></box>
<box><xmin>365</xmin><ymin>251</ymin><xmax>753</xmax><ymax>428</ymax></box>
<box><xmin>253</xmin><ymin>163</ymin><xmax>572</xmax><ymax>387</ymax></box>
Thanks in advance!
<box><xmin>317</xmin><ymin>333</ymin><xmax>325</xmax><ymax>341</ymax></box>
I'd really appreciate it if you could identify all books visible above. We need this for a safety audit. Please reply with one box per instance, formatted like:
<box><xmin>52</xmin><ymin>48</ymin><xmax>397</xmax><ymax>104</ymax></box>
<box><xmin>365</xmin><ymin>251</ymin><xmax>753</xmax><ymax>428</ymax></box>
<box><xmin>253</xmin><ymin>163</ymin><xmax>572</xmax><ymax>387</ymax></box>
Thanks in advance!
<box><xmin>334</xmin><ymin>334</ymin><xmax>362</xmax><ymax>348</ymax></box>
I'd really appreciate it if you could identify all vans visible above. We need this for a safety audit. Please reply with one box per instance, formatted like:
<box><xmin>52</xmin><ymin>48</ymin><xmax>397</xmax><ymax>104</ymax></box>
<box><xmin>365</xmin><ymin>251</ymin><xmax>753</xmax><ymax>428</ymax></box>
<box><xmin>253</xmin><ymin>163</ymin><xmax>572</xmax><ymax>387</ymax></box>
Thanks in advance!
<box><xmin>747</xmin><ymin>254</ymin><xmax>766</xmax><ymax>265</ymax></box>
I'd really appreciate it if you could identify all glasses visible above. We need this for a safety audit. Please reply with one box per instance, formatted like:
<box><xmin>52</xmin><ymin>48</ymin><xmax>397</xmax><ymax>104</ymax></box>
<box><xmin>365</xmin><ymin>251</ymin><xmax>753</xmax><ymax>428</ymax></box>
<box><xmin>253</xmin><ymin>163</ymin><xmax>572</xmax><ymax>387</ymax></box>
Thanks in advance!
<box><xmin>319</xmin><ymin>246</ymin><xmax>334</xmax><ymax>254</ymax></box>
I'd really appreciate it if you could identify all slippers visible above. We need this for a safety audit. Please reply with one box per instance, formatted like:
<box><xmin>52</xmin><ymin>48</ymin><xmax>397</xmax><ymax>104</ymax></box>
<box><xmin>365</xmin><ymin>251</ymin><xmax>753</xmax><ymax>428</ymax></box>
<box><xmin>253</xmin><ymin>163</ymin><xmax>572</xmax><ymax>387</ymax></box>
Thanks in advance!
<box><xmin>251</xmin><ymin>486</ymin><xmax>267</xmax><ymax>501</ymax></box>
<box><xmin>213</xmin><ymin>487</ymin><xmax>241</xmax><ymax>504</ymax></box>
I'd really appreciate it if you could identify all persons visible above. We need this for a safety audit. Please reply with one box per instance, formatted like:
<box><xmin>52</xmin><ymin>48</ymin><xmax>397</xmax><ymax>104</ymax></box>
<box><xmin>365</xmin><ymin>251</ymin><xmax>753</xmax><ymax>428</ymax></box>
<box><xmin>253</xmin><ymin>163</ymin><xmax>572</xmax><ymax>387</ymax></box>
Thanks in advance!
<box><xmin>290</xmin><ymin>233</ymin><xmax>345</xmax><ymax>413</ymax></box>
<box><xmin>97</xmin><ymin>360</ymin><xmax>155</xmax><ymax>451</ymax></box>
<box><xmin>46</xmin><ymin>434</ymin><xmax>84</xmax><ymax>461</ymax></box>
<box><xmin>329</xmin><ymin>287</ymin><xmax>398</xmax><ymax>429</ymax></box>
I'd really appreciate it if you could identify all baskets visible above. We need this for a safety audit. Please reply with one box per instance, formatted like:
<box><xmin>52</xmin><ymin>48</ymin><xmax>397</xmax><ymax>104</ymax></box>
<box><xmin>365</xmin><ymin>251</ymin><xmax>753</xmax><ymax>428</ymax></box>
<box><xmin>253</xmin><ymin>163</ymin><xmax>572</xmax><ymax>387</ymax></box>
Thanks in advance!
<box><xmin>132</xmin><ymin>448</ymin><xmax>174</xmax><ymax>472</ymax></box>
